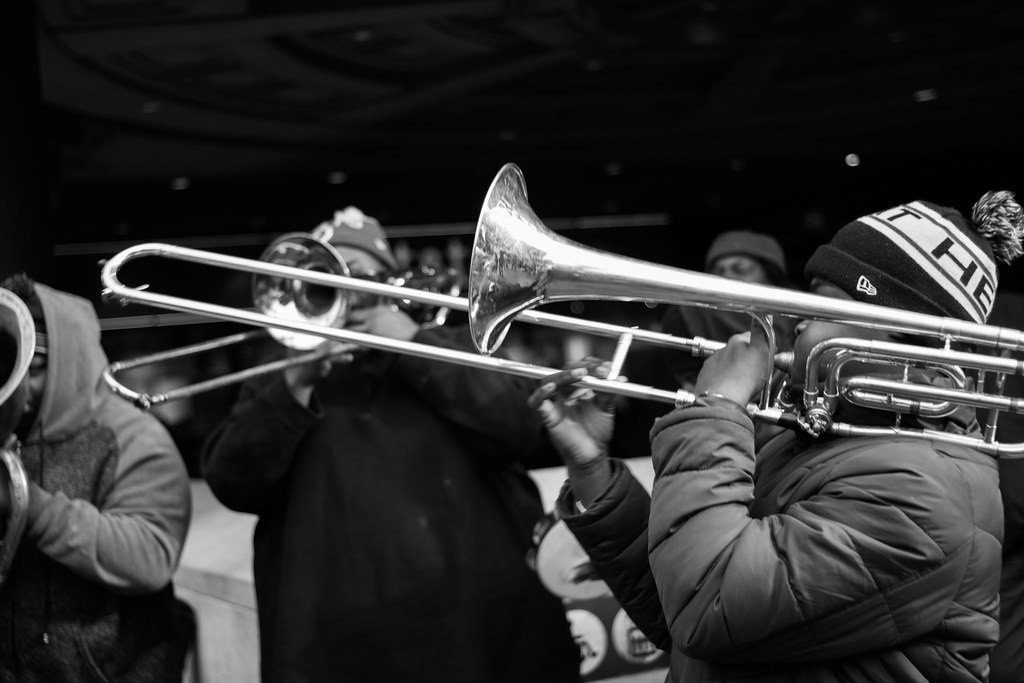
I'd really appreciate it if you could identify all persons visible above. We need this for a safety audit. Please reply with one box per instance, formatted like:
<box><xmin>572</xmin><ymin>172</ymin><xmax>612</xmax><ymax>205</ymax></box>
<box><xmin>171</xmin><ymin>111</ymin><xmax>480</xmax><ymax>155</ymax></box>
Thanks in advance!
<box><xmin>527</xmin><ymin>190</ymin><xmax>1024</xmax><ymax>683</ymax></box>
<box><xmin>603</xmin><ymin>229</ymin><xmax>788</xmax><ymax>460</ymax></box>
<box><xmin>202</xmin><ymin>206</ymin><xmax>582</xmax><ymax>683</ymax></box>
<box><xmin>0</xmin><ymin>277</ymin><xmax>192</xmax><ymax>683</ymax></box>
<box><xmin>973</xmin><ymin>295</ymin><xmax>1024</xmax><ymax>683</ymax></box>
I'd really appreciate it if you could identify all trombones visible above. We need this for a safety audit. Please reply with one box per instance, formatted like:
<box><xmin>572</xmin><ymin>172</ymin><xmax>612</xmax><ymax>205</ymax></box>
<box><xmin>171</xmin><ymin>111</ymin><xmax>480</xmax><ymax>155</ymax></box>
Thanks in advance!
<box><xmin>102</xmin><ymin>231</ymin><xmax>463</xmax><ymax>409</ymax></box>
<box><xmin>100</xmin><ymin>161</ymin><xmax>1018</xmax><ymax>459</ymax></box>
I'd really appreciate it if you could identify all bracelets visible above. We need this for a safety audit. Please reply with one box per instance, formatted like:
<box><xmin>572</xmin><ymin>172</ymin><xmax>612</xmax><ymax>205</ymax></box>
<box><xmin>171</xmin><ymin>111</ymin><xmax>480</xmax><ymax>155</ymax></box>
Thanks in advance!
<box><xmin>698</xmin><ymin>391</ymin><xmax>723</xmax><ymax>397</ymax></box>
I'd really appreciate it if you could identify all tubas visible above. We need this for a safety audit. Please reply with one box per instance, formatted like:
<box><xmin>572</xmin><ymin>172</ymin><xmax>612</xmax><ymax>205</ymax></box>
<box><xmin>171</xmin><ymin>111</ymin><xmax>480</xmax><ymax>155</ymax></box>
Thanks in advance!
<box><xmin>0</xmin><ymin>287</ymin><xmax>39</xmax><ymax>581</ymax></box>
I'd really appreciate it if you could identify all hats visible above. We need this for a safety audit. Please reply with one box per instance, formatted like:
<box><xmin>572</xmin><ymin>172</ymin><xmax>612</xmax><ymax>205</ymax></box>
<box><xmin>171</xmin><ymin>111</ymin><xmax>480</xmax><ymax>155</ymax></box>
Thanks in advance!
<box><xmin>311</xmin><ymin>206</ymin><xmax>399</xmax><ymax>272</ymax></box>
<box><xmin>803</xmin><ymin>191</ymin><xmax>1023</xmax><ymax>365</ymax></box>
<box><xmin>706</xmin><ymin>226</ymin><xmax>786</xmax><ymax>276</ymax></box>
<box><xmin>5</xmin><ymin>273</ymin><xmax>49</xmax><ymax>358</ymax></box>
